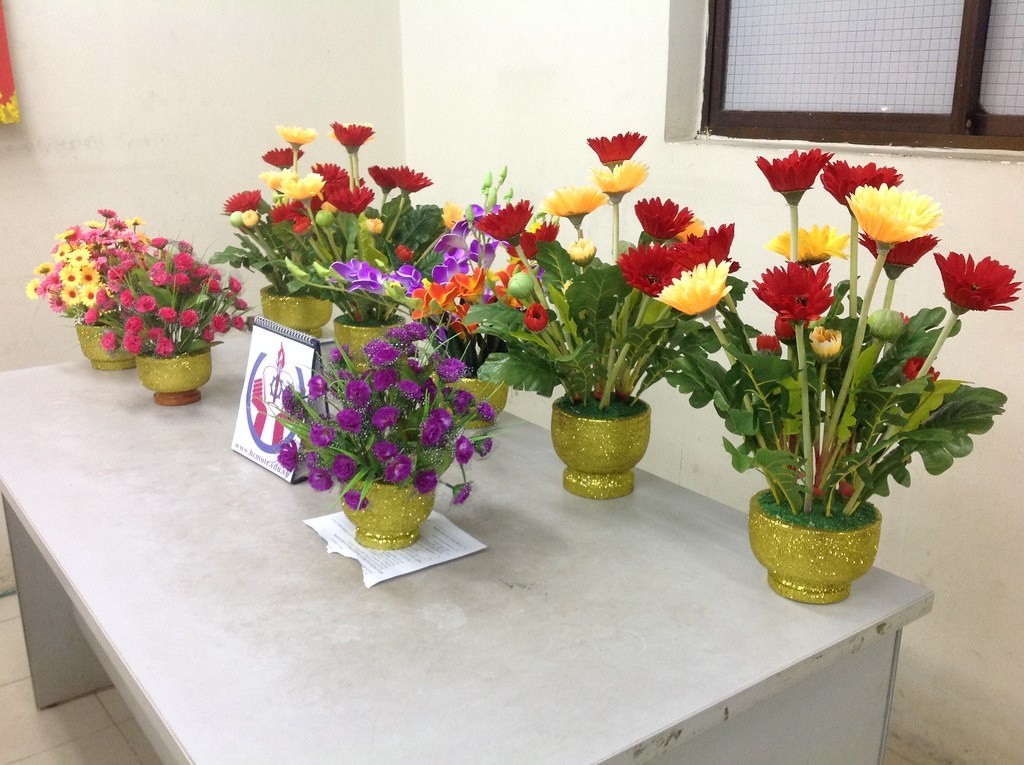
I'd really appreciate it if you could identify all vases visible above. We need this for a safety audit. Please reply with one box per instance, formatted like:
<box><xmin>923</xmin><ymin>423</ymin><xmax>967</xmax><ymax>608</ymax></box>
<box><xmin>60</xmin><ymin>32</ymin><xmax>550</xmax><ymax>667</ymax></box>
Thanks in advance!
<box><xmin>746</xmin><ymin>487</ymin><xmax>882</xmax><ymax>608</ymax></box>
<box><xmin>333</xmin><ymin>312</ymin><xmax>408</xmax><ymax>374</ymax></box>
<box><xmin>551</xmin><ymin>392</ymin><xmax>653</xmax><ymax>499</ymax></box>
<box><xmin>135</xmin><ymin>351</ymin><xmax>215</xmax><ymax>407</ymax></box>
<box><xmin>259</xmin><ymin>285</ymin><xmax>334</xmax><ymax>339</ymax></box>
<box><xmin>338</xmin><ymin>482</ymin><xmax>435</xmax><ymax>551</ymax></box>
<box><xmin>75</xmin><ymin>319</ymin><xmax>135</xmax><ymax>373</ymax></box>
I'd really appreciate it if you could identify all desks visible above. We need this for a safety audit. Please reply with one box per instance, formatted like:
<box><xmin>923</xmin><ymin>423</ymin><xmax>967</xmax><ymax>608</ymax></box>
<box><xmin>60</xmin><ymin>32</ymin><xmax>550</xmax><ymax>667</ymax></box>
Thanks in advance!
<box><xmin>3</xmin><ymin>323</ymin><xmax>935</xmax><ymax>764</ymax></box>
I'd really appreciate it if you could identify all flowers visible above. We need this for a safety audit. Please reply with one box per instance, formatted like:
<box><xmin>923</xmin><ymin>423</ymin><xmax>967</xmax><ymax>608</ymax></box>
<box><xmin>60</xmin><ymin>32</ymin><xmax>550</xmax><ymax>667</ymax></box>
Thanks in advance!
<box><xmin>262</xmin><ymin>322</ymin><xmax>500</xmax><ymax>513</ymax></box>
<box><xmin>618</xmin><ymin>147</ymin><xmax>1024</xmax><ymax>519</ymax></box>
<box><xmin>81</xmin><ymin>233</ymin><xmax>250</xmax><ymax>362</ymax></box>
<box><xmin>459</xmin><ymin>127</ymin><xmax>748</xmax><ymax>415</ymax></box>
<box><xmin>208</xmin><ymin>118</ymin><xmax>464</xmax><ymax>327</ymax></box>
<box><xmin>22</xmin><ymin>207</ymin><xmax>149</xmax><ymax>324</ymax></box>
